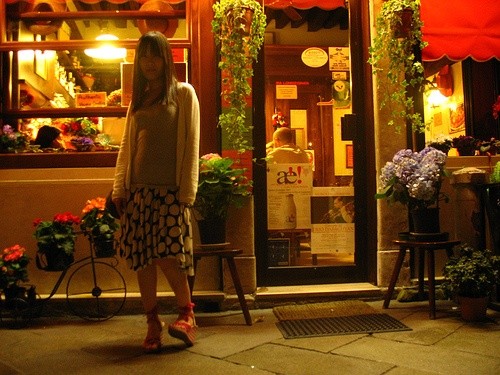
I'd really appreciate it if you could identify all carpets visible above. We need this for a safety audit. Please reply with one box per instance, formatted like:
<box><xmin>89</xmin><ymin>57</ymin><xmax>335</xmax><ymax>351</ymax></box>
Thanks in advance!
<box><xmin>272</xmin><ymin>299</ymin><xmax>413</xmax><ymax>340</ymax></box>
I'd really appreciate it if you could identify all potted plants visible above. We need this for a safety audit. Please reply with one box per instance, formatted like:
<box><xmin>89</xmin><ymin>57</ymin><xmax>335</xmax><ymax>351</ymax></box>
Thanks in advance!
<box><xmin>366</xmin><ymin>0</ymin><xmax>434</xmax><ymax>136</ymax></box>
<box><xmin>438</xmin><ymin>242</ymin><xmax>500</xmax><ymax>321</ymax></box>
<box><xmin>212</xmin><ymin>0</ymin><xmax>267</xmax><ymax>154</ymax></box>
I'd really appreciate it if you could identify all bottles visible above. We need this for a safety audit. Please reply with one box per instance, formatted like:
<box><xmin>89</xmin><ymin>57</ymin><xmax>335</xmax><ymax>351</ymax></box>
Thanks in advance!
<box><xmin>283</xmin><ymin>194</ymin><xmax>297</xmax><ymax>230</ymax></box>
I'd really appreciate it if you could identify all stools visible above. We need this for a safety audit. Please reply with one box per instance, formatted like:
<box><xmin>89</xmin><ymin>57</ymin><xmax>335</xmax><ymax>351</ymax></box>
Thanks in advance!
<box><xmin>187</xmin><ymin>250</ymin><xmax>253</xmax><ymax>325</ymax></box>
<box><xmin>383</xmin><ymin>240</ymin><xmax>460</xmax><ymax>320</ymax></box>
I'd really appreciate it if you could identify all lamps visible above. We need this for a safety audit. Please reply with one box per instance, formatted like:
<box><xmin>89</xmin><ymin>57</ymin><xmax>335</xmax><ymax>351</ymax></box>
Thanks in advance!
<box><xmin>428</xmin><ymin>61</ymin><xmax>453</xmax><ymax>104</ymax></box>
<box><xmin>83</xmin><ymin>25</ymin><xmax>127</xmax><ymax>59</ymax></box>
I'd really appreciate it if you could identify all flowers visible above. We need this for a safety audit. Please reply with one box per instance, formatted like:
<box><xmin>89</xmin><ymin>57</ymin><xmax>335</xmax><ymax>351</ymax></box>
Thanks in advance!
<box><xmin>193</xmin><ymin>151</ymin><xmax>255</xmax><ymax>220</ymax></box>
<box><xmin>0</xmin><ymin>195</ymin><xmax>120</xmax><ymax>286</ymax></box>
<box><xmin>0</xmin><ymin>124</ymin><xmax>16</xmax><ymax>147</ymax></box>
<box><xmin>96</xmin><ymin>240</ymin><xmax>113</xmax><ymax>258</ymax></box>
<box><xmin>491</xmin><ymin>95</ymin><xmax>500</xmax><ymax>120</ymax></box>
<box><xmin>374</xmin><ymin>147</ymin><xmax>447</xmax><ymax>207</ymax></box>
<box><xmin>452</xmin><ymin>135</ymin><xmax>479</xmax><ymax>148</ymax></box>
<box><xmin>60</xmin><ymin>117</ymin><xmax>100</xmax><ymax>144</ymax></box>
<box><xmin>425</xmin><ymin>135</ymin><xmax>452</xmax><ymax>149</ymax></box>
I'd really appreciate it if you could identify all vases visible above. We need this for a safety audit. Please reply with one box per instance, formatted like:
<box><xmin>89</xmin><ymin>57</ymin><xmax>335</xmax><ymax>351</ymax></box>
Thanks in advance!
<box><xmin>194</xmin><ymin>220</ymin><xmax>230</xmax><ymax>251</ymax></box>
<box><xmin>45</xmin><ymin>248</ymin><xmax>74</xmax><ymax>266</ymax></box>
<box><xmin>409</xmin><ymin>207</ymin><xmax>449</xmax><ymax>242</ymax></box>
<box><xmin>442</xmin><ymin>149</ymin><xmax>475</xmax><ymax>156</ymax></box>
<box><xmin>4</xmin><ymin>286</ymin><xmax>25</xmax><ymax>299</ymax></box>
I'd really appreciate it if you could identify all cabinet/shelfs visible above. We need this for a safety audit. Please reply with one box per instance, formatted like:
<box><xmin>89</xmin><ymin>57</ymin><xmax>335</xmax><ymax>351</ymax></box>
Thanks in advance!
<box><xmin>52</xmin><ymin>50</ymin><xmax>87</xmax><ymax>109</ymax></box>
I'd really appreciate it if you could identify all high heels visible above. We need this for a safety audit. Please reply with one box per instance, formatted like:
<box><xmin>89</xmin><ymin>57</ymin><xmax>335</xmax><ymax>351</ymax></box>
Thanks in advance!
<box><xmin>169</xmin><ymin>302</ymin><xmax>198</xmax><ymax>345</ymax></box>
<box><xmin>143</xmin><ymin>307</ymin><xmax>163</xmax><ymax>353</ymax></box>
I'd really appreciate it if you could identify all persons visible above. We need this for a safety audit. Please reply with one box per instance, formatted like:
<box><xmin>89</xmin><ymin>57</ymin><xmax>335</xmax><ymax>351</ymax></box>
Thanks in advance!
<box><xmin>30</xmin><ymin>124</ymin><xmax>66</xmax><ymax>150</ymax></box>
<box><xmin>266</xmin><ymin>127</ymin><xmax>310</xmax><ymax>163</ymax></box>
<box><xmin>112</xmin><ymin>30</ymin><xmax>201</xmax><ymax>353</ymax></box>
